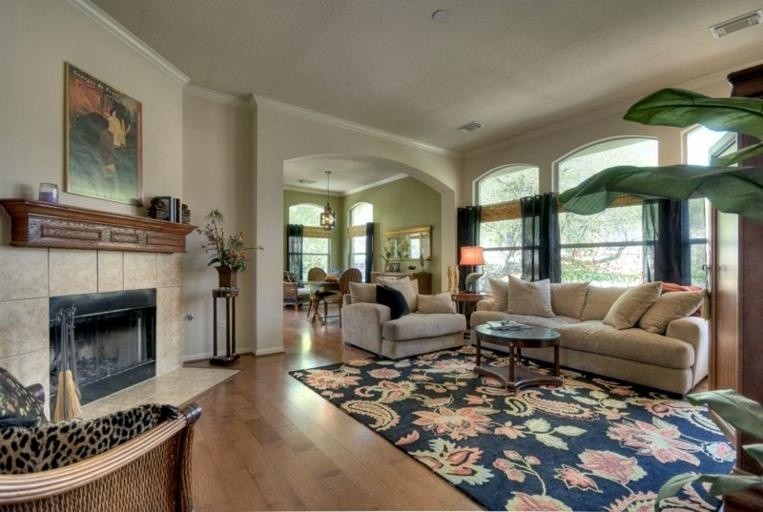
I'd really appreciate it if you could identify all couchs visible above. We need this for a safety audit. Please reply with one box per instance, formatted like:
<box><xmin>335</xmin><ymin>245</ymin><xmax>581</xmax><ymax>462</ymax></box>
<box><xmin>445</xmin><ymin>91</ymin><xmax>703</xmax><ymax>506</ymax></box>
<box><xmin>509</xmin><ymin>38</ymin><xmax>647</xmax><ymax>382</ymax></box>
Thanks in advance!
<box><xmin>341</xmin><ymin>294</ymin><xmax>467</xmax><ymax>360</ymax></box>
<box><xmin>470</xmin><ymin>289</ymin><xmax>709</xmax><ymax>401</ymax></box>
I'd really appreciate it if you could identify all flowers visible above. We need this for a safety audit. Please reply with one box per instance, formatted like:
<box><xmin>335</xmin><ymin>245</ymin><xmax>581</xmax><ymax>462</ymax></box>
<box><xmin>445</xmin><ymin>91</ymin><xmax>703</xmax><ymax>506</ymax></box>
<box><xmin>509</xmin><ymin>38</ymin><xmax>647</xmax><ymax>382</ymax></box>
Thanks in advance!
<box><xmin>196</xmin><ymin>208</ymin><xmax>263</xmax><ymax>272</ymax></box>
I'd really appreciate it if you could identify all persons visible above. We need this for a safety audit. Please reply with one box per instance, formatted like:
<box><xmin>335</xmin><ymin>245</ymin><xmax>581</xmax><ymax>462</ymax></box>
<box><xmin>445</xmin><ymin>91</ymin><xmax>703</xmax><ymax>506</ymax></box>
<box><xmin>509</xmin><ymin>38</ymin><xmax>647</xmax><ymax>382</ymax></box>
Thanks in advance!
<box><xmin>100</xmin><ymin>101</ymin><xmax>135</xmax><ymax>173</ymax></box>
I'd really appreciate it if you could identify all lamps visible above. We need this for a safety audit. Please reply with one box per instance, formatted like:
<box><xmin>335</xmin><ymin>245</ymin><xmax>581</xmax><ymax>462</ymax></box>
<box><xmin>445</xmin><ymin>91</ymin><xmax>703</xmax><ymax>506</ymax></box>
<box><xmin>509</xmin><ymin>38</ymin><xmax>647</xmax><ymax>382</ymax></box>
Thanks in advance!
<box><xmin>320</xmin><ymin>171</ymin><xmax>336</xmax><ymax>230</ymax></box>
<box><xmin>460</xmin><ymin>246</ymin><xmax>486</xmax><ymax>293</ymax></box>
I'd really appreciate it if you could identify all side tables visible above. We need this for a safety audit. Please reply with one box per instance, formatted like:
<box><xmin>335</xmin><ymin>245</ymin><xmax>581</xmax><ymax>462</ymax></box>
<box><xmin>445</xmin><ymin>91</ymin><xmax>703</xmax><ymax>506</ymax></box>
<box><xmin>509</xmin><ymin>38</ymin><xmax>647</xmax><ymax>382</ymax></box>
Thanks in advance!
<box><xmin>450</xmin><ymin>292</ymin><xmax>483</xmax><ymax>335</ymax></box>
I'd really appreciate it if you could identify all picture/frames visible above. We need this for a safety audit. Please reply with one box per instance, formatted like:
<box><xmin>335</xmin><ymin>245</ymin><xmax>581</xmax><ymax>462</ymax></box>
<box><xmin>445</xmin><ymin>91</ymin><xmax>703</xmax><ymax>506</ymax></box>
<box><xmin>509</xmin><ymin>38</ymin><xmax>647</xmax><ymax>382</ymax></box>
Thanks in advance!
<box><xmin>63</xmin><ymin>61</ymin><xmax>143</xmax><ymax>208</ymax></box>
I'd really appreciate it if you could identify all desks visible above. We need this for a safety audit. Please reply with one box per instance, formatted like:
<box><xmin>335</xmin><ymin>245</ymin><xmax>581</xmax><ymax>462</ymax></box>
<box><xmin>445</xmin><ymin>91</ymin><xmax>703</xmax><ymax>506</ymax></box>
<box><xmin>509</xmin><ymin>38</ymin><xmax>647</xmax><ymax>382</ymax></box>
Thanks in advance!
<box><xmin>371</xmin><ymin>271</ymin><xmax>432</xmax><ymax>295</ymax></box>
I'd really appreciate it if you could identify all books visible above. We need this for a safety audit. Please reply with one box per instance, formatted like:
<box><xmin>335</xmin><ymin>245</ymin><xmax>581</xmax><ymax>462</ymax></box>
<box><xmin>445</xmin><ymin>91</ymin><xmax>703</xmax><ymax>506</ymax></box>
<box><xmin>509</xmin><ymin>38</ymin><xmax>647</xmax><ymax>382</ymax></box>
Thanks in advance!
<box><xmin>155</xmin><ymin>196</ymin><xmax>182</xmax><ymax>223</ymax></box>
<box><xmin>486</xmin><ymin>320</ymin><xmax>523</xmax><ymax>331</ymax></box>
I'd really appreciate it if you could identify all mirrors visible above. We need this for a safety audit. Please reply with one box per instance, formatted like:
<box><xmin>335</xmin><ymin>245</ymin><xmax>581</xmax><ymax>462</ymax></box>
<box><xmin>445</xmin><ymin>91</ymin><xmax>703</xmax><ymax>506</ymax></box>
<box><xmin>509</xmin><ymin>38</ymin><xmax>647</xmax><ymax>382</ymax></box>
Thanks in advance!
<box><xmin>384</xmin><ymin>226</ymin><xmax>432</xmax><ymax>262</ymax></box>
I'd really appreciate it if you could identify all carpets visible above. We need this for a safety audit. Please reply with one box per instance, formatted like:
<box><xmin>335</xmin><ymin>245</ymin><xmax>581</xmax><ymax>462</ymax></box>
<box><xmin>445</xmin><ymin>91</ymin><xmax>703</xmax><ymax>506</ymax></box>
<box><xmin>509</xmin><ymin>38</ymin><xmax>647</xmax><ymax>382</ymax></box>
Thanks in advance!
<box><xmin>288</xmin><ymin>344</ymin><xmax>737</xmax><ymax>511</ymax></box>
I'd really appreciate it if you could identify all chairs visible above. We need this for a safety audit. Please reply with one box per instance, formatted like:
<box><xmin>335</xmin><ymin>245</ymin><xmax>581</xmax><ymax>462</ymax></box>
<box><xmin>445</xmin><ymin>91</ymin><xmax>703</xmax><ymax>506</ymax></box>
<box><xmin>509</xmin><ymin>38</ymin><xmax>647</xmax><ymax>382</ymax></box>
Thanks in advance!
<box><xmin>0</xmin><ymin>366</ymin><xmax>202</xmax><ymax>512</ymax></box>
<box><xmin>283</xmin><ymin>267</ymin><xmax>362</xmax><ymax>328</ymax></box>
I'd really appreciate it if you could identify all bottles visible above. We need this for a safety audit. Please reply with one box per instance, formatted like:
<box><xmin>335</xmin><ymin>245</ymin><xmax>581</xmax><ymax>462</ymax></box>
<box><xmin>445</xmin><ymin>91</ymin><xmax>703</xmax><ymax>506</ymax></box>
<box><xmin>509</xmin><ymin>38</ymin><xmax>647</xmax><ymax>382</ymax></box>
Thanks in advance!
<box><xmin>38</xmin><ymin>183</ymin><xmax>60</xmax><ymax>206</ymax></box>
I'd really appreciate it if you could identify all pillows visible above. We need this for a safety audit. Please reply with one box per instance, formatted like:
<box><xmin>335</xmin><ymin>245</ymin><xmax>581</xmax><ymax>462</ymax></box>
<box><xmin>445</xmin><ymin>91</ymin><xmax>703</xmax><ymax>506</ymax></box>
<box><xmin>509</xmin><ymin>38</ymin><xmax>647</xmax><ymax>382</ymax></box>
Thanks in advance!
<box><xmin>349</xmin><ymin>276</ymin><xmax>456</xmax><ymax>320</ymax></box>
<box><xmin>489</xmin><ymin>274</ymin><xmax>703</xmax><ymax>334</ymax></box>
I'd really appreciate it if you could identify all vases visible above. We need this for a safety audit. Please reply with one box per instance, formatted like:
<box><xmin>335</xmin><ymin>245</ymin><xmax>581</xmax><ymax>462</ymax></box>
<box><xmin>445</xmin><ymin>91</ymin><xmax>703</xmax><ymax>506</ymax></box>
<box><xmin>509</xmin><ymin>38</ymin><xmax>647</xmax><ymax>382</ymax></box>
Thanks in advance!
<box><xmin>214</xmin><ymin>266</ymin><xmax>240</xmax><ymax>290</ymax></box>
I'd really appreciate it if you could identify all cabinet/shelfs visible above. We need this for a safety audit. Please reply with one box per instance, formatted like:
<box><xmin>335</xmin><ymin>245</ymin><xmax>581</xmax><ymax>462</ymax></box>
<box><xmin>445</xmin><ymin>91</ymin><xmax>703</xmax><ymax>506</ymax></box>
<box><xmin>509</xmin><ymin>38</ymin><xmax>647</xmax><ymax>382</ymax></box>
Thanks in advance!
<box><xmin>209</xmin><ymin>289</ymin><xmax>240</xmax><ymax>366</ymax></box>
